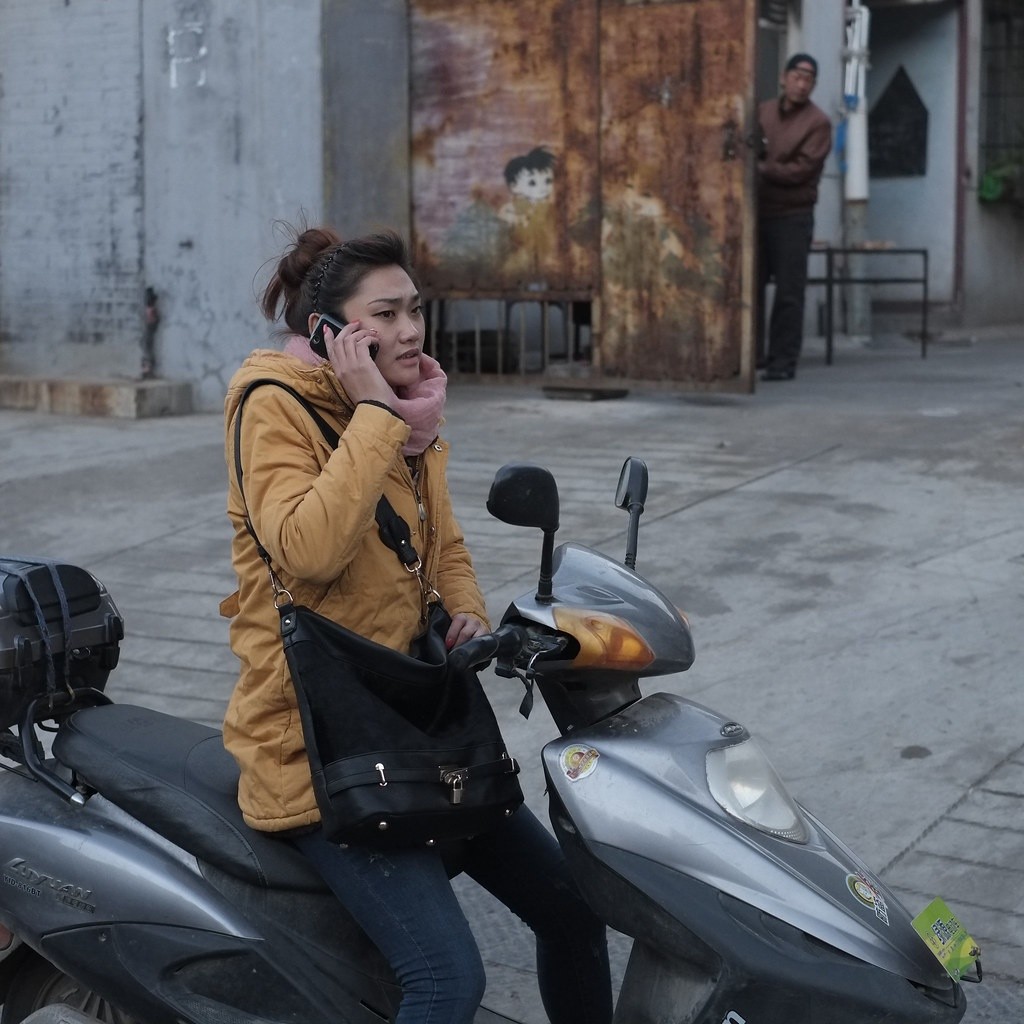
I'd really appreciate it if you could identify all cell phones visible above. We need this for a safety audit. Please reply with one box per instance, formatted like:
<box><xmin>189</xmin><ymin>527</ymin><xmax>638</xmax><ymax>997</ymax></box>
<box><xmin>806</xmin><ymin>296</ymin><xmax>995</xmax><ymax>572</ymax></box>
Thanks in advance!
<box><xmin>309</xmin><ymin>313</ymin><xmax>379</xmax><ymax>365</ymax></box>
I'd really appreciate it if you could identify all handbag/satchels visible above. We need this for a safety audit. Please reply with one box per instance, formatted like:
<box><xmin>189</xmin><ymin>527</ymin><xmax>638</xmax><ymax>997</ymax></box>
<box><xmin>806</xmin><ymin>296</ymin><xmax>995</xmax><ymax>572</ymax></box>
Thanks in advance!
<box><xmin>278</xmin><ymin>601</ymin><xmax>530</xmax><ymax>856</ymax></box>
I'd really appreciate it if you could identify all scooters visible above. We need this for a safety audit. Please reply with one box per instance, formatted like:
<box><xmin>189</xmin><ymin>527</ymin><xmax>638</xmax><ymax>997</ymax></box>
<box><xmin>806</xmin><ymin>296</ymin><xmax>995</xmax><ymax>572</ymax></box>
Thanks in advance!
<box><xmin>0</xmin><ymin>455</ymin><xmax>983</xmax><ymax>1023</ymax></box>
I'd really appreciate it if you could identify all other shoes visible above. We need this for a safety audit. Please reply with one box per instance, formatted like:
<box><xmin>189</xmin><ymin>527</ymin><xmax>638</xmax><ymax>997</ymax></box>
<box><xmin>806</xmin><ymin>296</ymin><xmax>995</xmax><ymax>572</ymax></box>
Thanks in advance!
<box><xmin>763</xmin><ymin>369</ymin><xmax>794</xmax><ymax>382</ymax></box>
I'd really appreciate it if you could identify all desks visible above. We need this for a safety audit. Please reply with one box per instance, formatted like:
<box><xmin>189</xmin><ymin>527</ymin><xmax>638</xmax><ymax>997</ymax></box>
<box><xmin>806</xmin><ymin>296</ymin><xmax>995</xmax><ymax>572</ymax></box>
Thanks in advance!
<box><xmin>771</xmin><ymin>248</ymin><xmax>927</xmax><ymax>364</ymax></box>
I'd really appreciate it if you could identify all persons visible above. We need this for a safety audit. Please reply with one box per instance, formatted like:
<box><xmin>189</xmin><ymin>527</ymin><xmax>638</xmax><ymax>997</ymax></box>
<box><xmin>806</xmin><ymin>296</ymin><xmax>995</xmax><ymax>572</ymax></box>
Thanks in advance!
<box><xmin>758</xmin><ymin>52</ymin><xmax>832</xmax><ymax>380</ymax></box>
<box><xmin>223</xmin><ymin>230</ymin><xmax>612</xmax><ymax>1023</ymax></box>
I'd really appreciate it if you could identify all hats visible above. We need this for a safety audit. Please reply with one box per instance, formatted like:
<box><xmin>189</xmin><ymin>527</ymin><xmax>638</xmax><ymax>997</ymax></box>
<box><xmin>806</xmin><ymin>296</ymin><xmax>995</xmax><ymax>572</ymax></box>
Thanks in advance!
<box><xmin>786</xmin><ymin>54</ymin><xmax>818</xmax><ymax>80</ymax></box>
